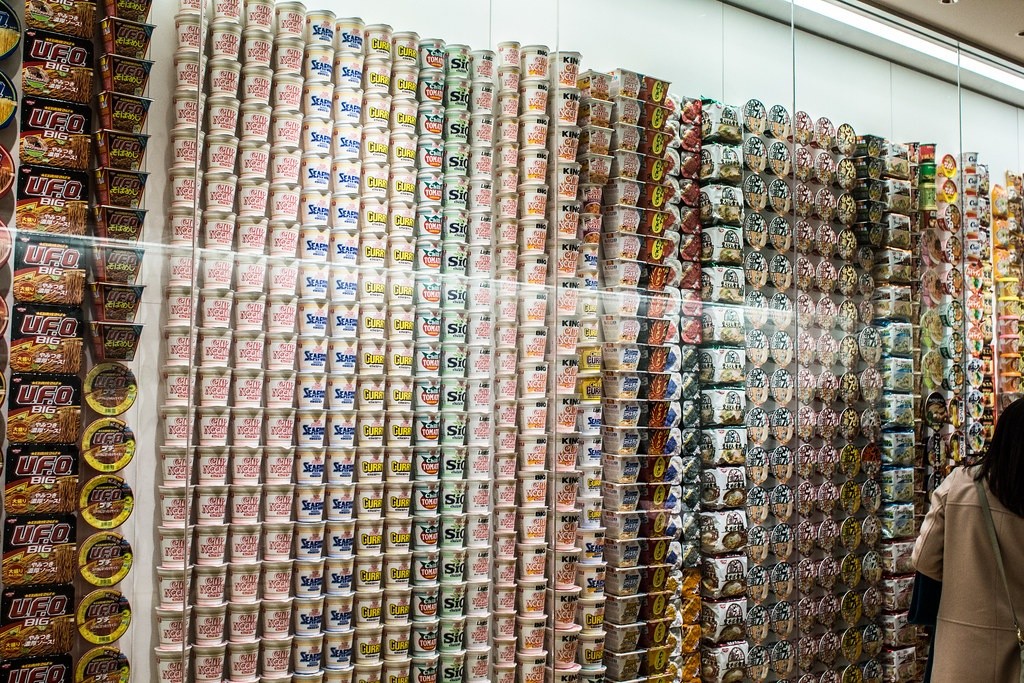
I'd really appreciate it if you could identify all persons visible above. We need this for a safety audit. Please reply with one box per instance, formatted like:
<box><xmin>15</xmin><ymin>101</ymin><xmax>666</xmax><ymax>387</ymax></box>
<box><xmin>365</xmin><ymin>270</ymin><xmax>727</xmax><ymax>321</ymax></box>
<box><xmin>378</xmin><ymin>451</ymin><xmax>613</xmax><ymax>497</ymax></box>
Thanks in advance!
<box><xmin>907</xmin><ymin>397</ymin><xmax>1024</xmax><ymax>683</ymax></box>
